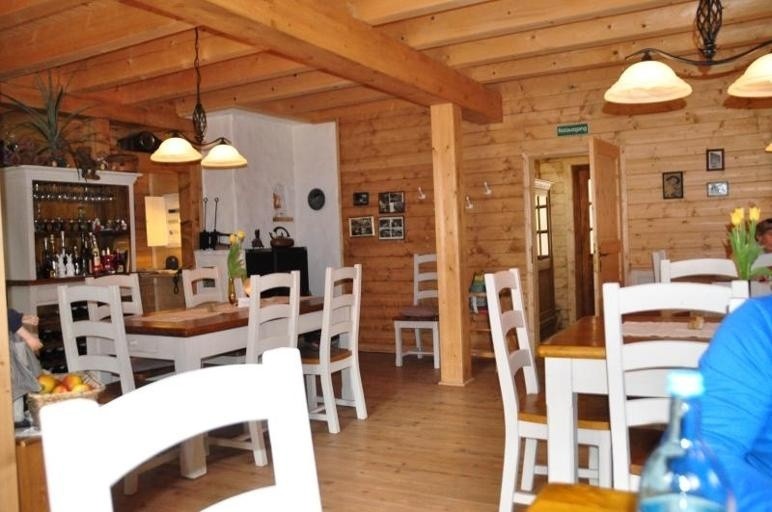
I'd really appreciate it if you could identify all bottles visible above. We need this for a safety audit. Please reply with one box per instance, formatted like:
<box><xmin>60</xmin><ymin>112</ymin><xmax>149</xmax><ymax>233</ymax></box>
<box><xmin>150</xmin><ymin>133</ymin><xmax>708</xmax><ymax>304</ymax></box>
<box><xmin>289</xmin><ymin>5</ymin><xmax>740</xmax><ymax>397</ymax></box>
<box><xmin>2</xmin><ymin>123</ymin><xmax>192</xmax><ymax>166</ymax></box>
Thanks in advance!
<box><xmin>36</xmin><ymin>230</ymin><xmax>126</xmax><ymax>278</ymax></box>
<box><xmin>636</xmin><ymin>370</ymin><xmax>733</xmax><ymax>512</ymax></box>
<box><xmin>34</xmin><ymin>182</ymin><xmax>115</xmax><ymax>202</ymax></box>
<box><xmin>35</xmin><ymin>203</ymin><xmax>128</xmax><ymax>233</ymax></box>
<box><xmin>39</xmin><ymin>305</ymin><xmax>88</xmax><ymax>373</ymax></box>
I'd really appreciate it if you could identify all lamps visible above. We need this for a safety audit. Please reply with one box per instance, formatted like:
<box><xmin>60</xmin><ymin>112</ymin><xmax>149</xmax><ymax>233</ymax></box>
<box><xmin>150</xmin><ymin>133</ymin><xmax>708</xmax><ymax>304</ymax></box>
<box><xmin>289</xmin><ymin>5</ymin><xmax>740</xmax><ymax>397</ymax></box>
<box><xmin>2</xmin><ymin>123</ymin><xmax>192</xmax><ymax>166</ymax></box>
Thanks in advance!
<box><xmin>150</xmin><ymin>27</ymin><xmax>248</xmax><ymax>169</ymax></box>
<box><xmin>604</xmin><ymin>0</ymin><xmax>771</xmax><ymax>104</ymax></box>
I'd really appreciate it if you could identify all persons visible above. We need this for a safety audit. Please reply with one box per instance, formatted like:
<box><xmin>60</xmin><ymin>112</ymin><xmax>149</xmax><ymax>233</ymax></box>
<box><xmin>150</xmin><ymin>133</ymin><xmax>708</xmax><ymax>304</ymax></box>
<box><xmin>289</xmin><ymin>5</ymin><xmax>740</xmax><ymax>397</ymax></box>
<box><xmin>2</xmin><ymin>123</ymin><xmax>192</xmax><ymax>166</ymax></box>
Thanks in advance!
<box><xmin>8</xmin><ymin>307</ymin><xmax>44</xmax><ymax>352</ymax></box>
<box><xmin>751</xmin><ymin>218</ymin><xmax>772</xmax><ymax>281</ymax></box>
<box><xmin>660</xmin><ymin>294</ymin><xmax>772</xmax><ymax>512</ymax></box>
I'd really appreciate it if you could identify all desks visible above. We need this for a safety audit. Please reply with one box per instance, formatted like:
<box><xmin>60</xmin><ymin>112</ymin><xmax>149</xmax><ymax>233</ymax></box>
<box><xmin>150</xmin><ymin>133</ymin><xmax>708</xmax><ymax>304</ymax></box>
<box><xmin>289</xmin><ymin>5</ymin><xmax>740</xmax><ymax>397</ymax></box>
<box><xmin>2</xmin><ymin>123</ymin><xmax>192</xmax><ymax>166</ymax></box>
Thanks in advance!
<box><xmin>15</xmin><ymin>396</ymin><xmax>114</xmax><ymax>511</ymax></box>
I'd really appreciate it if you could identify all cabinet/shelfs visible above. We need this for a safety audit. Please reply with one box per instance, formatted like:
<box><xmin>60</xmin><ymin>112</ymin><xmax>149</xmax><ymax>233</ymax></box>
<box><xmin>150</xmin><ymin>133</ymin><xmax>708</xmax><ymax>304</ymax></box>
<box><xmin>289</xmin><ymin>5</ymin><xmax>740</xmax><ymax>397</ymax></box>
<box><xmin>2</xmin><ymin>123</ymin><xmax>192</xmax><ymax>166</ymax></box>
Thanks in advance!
<box><xmin>3</xmin><ymin>165</ymin><xmax>145</xmax><ymax>335</ymax></box>
<box><xmin>469</xmin><ymin>293</ymin><xmax>498</xmax><ymax>372</ymax></box>
<box><xmin>246</xmin><ymin>246</ymin><xmax>308</xmax><ymax>297</ymax></box>
<box><xmin>194</xmin><ymin>249</ymin><xmax>246</xmax><ymax>298</ymax></box>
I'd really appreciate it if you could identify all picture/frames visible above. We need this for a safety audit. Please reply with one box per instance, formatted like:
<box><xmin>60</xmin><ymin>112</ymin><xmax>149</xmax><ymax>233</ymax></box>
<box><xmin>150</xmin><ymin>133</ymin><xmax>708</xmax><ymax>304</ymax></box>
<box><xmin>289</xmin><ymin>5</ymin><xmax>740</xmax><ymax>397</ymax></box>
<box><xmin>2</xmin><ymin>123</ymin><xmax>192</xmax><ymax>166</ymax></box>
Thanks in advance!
<box><xmin>378</xmin><ymin>216</ymin><xmax>405</xmax><ymax>239</ymax></box>
<box><xmin>706</xmin><ymin>180</ymin><xmax>729</xmax><ymax>197</ymax></box>
<box><xmin>378</xmin><ymin>190</ymin><xmax>405</xmax><ymax>213</ymax></box>
<box><xmin>663</xmin><ymin>171</ymin><xmax>683</xmax><ymax>199</ymax></box>
<box><xmin>348</xmin><ymin>216</ymin><xmax>375</xmax><ymax>237</ymax></box>
<box><xmin>352</xmin><ymin>191</ymin><xmax>369</xmax><ymax>206</ymax></box>
<box><xmin>707</xmin><ymin>149</ymin><xmax>725</xmax><ymax>170</ymax></box>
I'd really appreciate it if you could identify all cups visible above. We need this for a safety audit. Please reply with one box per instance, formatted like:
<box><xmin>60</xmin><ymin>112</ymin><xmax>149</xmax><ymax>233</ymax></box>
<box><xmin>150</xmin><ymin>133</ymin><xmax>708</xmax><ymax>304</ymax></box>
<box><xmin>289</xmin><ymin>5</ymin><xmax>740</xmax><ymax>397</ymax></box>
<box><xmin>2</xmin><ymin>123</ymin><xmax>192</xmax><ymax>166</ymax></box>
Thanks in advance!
<box><xmin>687</xmin><ymin>311</ymin><xmax>704</xmax><ymax>329</ymax></box>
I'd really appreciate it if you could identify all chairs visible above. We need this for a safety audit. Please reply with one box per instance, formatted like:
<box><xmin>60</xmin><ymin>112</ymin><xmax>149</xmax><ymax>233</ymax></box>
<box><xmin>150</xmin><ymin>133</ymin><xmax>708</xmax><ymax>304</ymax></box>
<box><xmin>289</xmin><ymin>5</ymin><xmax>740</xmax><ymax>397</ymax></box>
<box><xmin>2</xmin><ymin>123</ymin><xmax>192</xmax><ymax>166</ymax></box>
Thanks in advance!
<box><xmin>392</xmin><ymin>252</ymin><xmax>440</xmax><ymax>369</ymax></box>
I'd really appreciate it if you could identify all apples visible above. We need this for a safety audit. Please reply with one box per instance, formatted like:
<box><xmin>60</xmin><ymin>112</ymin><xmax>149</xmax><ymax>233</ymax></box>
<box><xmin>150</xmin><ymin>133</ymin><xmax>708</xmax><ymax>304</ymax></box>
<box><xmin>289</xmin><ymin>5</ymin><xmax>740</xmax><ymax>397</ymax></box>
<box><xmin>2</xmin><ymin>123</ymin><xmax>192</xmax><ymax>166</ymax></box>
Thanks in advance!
<box><xmin>35</xmin><ymin>374</ymin><xmax>90</xmax><ymax>396</ymax></box>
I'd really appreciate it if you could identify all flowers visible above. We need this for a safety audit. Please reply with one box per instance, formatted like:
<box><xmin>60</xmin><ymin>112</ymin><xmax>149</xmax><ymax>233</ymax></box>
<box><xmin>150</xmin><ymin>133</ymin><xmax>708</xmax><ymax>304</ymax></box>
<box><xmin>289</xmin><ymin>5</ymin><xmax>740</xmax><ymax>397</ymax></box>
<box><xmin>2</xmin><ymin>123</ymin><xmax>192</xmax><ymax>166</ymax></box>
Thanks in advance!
<box><xmin>726</xmin><ymin>204</ymin><xmax>771</xmax><ymax>281</ymax></box>
<box><xmin>224</xmin><ymin>230</ymin><xmax>247</xmax><ymax>280</ymax></box>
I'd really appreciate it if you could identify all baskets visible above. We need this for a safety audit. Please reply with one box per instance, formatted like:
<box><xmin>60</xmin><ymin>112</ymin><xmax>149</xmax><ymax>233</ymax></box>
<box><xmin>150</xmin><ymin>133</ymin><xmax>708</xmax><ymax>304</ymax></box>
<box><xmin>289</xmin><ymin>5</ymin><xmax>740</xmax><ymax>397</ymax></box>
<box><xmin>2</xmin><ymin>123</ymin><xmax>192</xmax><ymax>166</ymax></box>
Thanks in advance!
<box><xmin>24</xmin><ymin>371</ymin><xmax>107</xmax><ymax>428</ymax></box>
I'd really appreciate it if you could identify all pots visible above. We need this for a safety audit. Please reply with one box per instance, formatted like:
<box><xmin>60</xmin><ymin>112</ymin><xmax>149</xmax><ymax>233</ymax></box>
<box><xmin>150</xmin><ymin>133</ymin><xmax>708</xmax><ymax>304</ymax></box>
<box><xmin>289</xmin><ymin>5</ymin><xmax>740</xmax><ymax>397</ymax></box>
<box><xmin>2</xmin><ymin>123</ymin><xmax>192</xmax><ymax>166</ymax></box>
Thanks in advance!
<box><xmin>107</xmin><ymin>155</ymin><xmax>138</xmax><ymax>172</ymax></box>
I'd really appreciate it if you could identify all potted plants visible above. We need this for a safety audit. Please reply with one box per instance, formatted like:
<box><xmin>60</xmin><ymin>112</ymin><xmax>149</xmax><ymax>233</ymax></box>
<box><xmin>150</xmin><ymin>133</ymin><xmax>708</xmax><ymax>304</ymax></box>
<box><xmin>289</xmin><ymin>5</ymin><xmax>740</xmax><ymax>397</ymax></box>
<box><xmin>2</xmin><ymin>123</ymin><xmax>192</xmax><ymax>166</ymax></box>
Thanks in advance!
<box><xmin>2</xmin><ymin>72</ymin><xmax>94</xmax><ymax>180</ymax></box>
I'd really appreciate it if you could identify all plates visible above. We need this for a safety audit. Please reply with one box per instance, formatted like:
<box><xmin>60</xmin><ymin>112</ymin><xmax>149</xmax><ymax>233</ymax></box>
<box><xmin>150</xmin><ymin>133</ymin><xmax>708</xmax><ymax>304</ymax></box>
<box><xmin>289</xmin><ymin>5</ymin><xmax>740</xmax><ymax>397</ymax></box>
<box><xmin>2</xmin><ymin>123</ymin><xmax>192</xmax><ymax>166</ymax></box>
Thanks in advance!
<box><xmin>308</xmin><ymin>189</ymin><xmax>324</xmax><ymax>210</ymax></box>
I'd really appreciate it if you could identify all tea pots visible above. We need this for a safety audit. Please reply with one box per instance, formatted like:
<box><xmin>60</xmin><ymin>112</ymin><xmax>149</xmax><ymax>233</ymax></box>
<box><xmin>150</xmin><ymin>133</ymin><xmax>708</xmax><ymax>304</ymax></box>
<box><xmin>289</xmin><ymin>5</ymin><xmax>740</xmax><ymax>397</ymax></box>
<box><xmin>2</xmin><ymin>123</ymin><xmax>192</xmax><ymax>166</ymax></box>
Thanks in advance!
<box><xmin>252</xmin><ymin>229</ymin><xmax>262</xmax><ymax>247</ymax></box>
<box><xmin>269</xmin><ymin>226</ymin><xmax>294</xmax><ymax>247</ymax></box>
